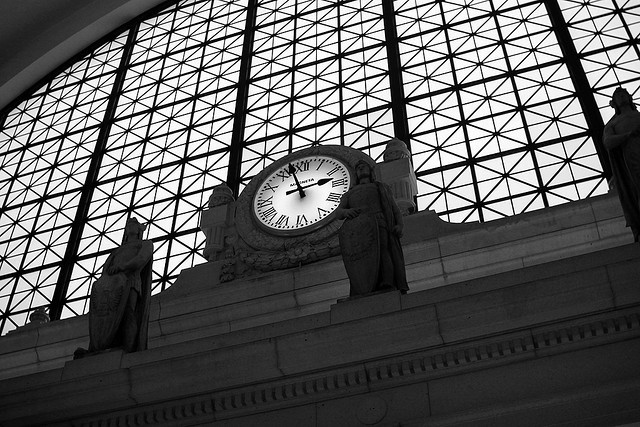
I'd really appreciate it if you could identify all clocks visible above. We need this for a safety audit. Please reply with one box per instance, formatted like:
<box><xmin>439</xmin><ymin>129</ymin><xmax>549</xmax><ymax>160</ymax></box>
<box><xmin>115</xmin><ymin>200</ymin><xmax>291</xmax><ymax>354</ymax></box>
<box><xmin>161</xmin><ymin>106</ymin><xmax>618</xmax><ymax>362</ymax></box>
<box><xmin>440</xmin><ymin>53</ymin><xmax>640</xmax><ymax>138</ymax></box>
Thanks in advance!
<box><xmin>237</xmin><ymin>143</ymin><xmax>376</xmax><ymax>250</ymax></box>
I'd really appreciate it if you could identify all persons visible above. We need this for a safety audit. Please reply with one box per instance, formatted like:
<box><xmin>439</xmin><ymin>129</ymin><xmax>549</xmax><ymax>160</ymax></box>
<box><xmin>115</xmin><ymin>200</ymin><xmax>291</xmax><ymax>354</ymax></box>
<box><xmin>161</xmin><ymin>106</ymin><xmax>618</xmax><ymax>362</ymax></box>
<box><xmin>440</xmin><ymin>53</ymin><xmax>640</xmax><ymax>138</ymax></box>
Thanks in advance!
<box><xmin>72</xmin><ymin>217</ymin><xmax>153</xmax><ymax>355</ymax></box>
<box><xmin>332</xmin><ymin>158</ymin><xmax>410</xmax><ymax>297</ymax></box>
<box><xmin>600</xmin><ymin>83</ymin><xmax>640</xmax><ymax>244</ymax></box>
